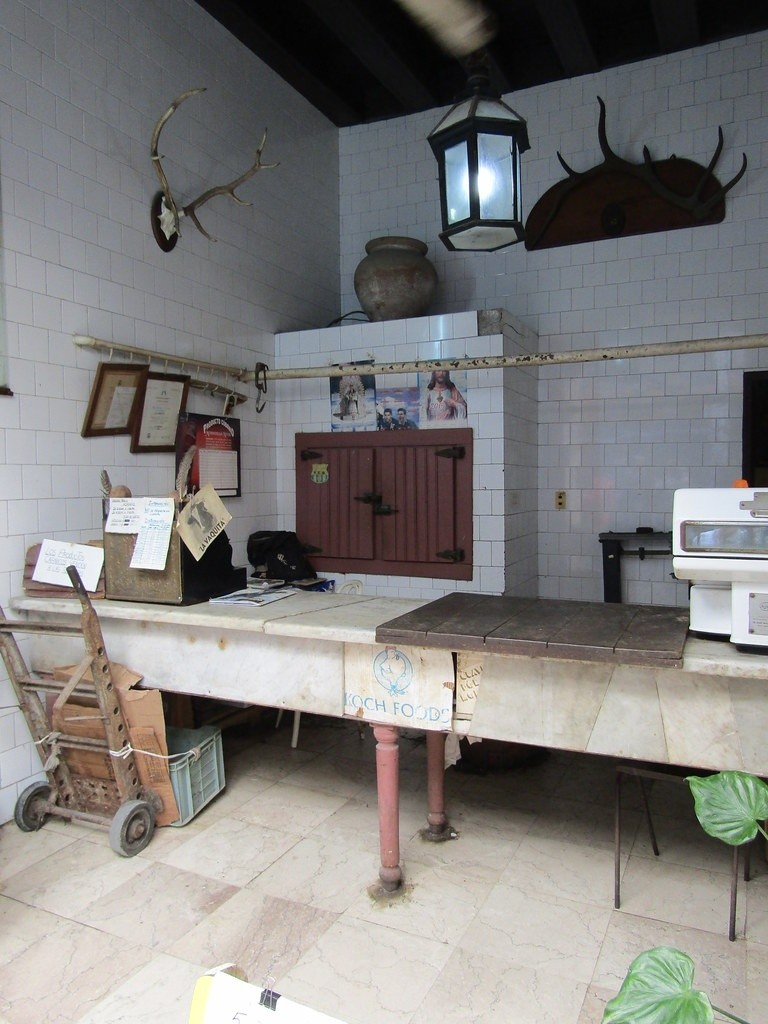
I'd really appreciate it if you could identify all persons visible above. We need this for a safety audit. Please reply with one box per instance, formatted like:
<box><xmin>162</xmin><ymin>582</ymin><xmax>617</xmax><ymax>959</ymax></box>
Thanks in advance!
<box><xmin>379</xmin><ymin>407</ymin><xmax>418</xmax><ymax>431</ymax></box>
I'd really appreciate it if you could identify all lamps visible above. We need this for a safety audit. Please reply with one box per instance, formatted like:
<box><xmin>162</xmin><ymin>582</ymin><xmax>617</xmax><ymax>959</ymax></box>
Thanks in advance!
<box><xmin>426</xmin><ymin>71</ymin><xmax>532</xmax><ymax>255</ymax></box>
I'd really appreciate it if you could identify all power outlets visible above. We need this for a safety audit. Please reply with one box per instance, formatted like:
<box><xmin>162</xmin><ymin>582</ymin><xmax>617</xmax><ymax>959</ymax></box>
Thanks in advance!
<box><xmin>555</xmin><ymin>491</ymin><xmax>566</xmax><ymax>509</ymax></box>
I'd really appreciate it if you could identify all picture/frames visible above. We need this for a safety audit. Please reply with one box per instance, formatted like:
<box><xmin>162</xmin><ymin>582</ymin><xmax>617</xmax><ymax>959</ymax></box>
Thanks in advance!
<box><xmin>129</xmin><ymin>371</ymin><xmax>192</xmax><ymax>452</ymax></box>
<box><xmin>81</xmin><ymin>361</ymin><xmax>151</xmax><ymax>437</ymax></box>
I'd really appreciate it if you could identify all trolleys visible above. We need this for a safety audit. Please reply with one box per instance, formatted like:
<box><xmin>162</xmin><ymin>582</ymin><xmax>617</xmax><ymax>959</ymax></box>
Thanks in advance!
<box><xmin>1</xmin><ymin>565</ymin><xmax>166</xmax><ymax>858</ymax></box>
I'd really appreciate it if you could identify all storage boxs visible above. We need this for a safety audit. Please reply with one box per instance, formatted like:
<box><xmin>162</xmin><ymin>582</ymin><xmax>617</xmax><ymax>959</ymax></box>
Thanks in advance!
<box><xmin>165</xmin><ymin>724</ymin><xmax>227</xmax><ymax>827</ymax></box>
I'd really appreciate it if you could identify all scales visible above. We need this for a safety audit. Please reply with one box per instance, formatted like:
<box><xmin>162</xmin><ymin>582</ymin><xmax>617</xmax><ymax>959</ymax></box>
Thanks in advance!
<box><xmin>598</xmin><ymin>526</ymin><xmax>678</xmax><ymax>603</ymax></box>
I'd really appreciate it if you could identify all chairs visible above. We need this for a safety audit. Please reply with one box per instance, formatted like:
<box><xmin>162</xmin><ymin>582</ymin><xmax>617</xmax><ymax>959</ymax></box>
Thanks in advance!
<box><xmin>612</xmin><ymin>760</ymin><xmax>752</xmax><ymax>941</ymax></box>
<box><xmin>274</xmin><ymin>579</ymin><xmax>364</xmax><ymax>749</ymax></box>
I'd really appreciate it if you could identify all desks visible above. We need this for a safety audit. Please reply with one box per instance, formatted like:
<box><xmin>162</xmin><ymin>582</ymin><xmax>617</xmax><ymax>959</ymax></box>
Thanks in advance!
<box><xmin>9</xmin><ymin>591</ymin><xmax>768</xmax><ymax>891</ymax></box>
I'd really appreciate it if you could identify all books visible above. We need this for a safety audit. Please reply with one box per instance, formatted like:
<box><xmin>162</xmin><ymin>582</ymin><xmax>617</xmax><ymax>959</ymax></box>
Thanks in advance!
<box><xmin>209</xmin><ymin>577</ymin><xmax>296</xmax><ymax>608</ymax></box>
